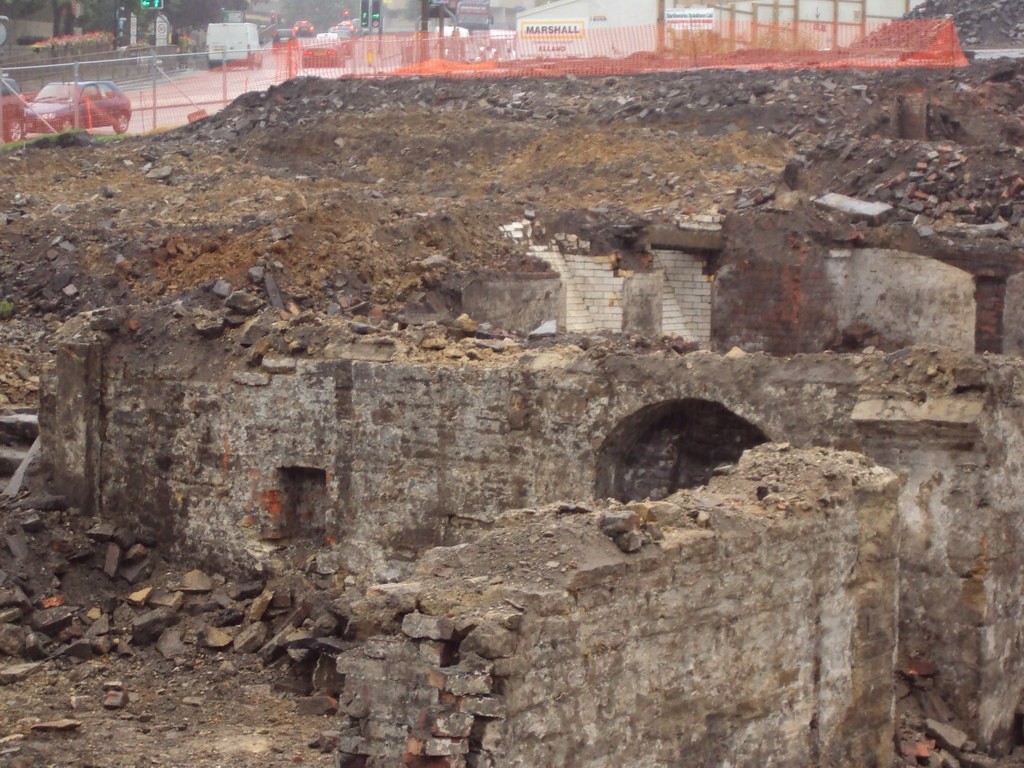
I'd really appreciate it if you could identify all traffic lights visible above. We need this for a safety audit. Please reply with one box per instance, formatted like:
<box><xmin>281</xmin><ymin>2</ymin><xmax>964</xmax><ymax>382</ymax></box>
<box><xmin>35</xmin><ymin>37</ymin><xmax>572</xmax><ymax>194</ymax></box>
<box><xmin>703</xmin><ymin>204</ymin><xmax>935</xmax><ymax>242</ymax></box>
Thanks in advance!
<box><xmin>140</xmin><ymin>0</ymin><xmax>163</xmax><ymax>10</ymax></box>
<box><xmin>360</xmin><ymin>0</ymin><xmax>368</xmax><ymax>27</ymax></box>
<box><xmin>270</xmin><ymin>13</ymin><xmax>275</xmax><ymax>24</ymax></box>
<box><xmin>372</xmin><ymin>0</ymin><xmax>381</xmax><ymax>29</ymax></box>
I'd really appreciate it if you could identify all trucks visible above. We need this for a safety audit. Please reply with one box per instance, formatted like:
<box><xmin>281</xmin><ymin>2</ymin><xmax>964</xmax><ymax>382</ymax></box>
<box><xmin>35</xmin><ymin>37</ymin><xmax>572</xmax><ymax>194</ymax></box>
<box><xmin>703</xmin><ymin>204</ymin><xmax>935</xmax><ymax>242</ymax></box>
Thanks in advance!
<box><xmin>206</xmin><ymin>23</ymin><xmax>263</xmax><ymax>69</ymax></box>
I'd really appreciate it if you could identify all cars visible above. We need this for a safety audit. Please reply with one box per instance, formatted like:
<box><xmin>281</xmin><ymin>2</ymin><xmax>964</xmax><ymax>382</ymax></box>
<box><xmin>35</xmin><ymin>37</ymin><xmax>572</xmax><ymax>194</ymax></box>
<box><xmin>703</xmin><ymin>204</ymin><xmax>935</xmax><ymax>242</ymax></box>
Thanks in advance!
<box><xmin>293</xmin><ymin>20</ymin><xmax>315</xmax><ymax>37</ymax></box>
<box><xmin>329</xmin><ymin>18</ymin><xmax>362</xmax><ymax>47</ymax></box>
<box><xmin>315</xmin><ymin>33</ymin><xmax>341</xmax><ymax>51</ymax></box>
<box><xmin>0</xmin><ymin>77</ymin><xmax>26</xmax><ymax>142</ymax></box>
<box><xmin>302</xmin><ymin>45</ymin><xmax>346</xmax><ymax>66</ymax></box>
<box><xmin>272</xmin><ymin>28</ymin><xmax>298</xmax><ymax>47</ymax></box>
<box><xmin>24</xmin><ymin>81</ymin><xmax>133</xmax><ymax>134</ymax></box>
